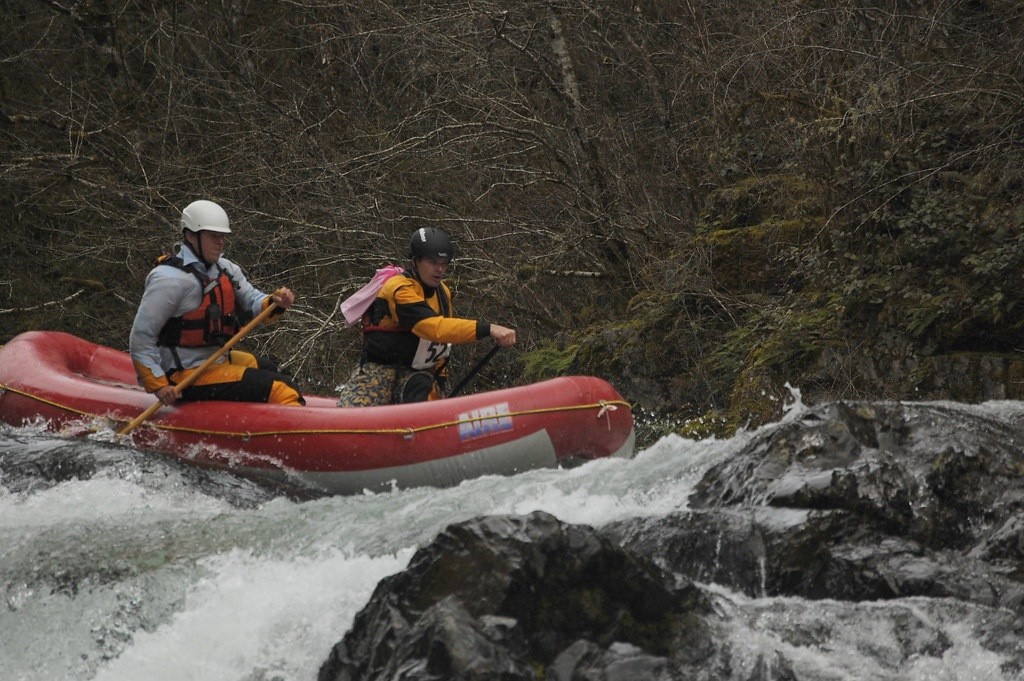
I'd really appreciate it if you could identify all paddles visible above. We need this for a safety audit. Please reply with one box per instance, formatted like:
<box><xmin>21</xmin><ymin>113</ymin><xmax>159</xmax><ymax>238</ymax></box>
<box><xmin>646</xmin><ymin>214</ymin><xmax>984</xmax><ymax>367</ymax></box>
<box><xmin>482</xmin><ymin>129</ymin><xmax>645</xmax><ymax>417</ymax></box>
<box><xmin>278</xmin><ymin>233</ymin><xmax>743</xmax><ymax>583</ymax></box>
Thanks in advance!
<box><xmin>117</xmin><ymin>288</ymin><xmax>300</xmax><ymax>438</ymax></box>
<box><xmin>448</xmin><ymin>342</ymin><xmax>503</xmax><ymax>398</ymax></box>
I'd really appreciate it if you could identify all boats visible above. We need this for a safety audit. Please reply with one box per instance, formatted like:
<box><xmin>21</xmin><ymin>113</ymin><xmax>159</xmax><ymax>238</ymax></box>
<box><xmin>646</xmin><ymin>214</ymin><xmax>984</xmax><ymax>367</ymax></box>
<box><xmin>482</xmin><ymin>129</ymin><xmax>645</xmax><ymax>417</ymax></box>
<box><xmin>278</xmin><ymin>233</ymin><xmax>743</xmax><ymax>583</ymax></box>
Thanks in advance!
<box><xmin>0</xmin><ymin>329</ymin><xmax>636</xmax><ymax>502</ymax></box>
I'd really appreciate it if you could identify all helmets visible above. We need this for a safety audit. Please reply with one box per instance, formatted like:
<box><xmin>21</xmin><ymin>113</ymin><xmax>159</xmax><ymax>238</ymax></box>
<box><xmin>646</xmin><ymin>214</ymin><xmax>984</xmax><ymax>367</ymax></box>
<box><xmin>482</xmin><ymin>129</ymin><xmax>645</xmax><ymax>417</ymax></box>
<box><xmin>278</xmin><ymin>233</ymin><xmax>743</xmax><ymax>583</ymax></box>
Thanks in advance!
<box><xmin>180</xmin><ymin>200</ymin><xmax>233</xmax><ymax>233</ymax></box>
<box><xmin>410</xmin><ymin>227</ymin><xmax>454</xmax><ymax>258</ymax></box>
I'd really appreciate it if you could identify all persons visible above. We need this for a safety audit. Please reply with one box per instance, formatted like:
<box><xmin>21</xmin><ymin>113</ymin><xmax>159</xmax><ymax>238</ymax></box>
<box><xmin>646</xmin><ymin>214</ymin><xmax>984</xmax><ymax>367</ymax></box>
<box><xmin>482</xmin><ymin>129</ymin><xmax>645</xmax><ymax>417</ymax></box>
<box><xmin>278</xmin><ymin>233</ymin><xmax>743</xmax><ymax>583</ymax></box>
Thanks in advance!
<box><xmin>336</xmin><ymin>227</ymin><xmax>516</xmax><ymax>408</ymax></box>
<box><xmin>129</xmin><ymin>199</ymin><xmax>307</xmax><ymax>408</ymax></box>
